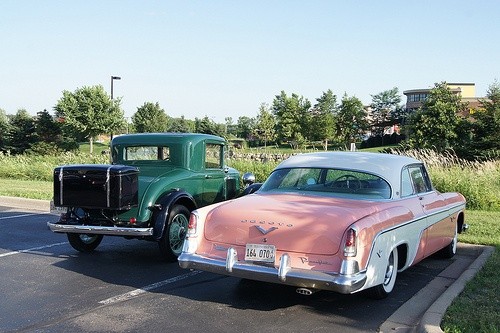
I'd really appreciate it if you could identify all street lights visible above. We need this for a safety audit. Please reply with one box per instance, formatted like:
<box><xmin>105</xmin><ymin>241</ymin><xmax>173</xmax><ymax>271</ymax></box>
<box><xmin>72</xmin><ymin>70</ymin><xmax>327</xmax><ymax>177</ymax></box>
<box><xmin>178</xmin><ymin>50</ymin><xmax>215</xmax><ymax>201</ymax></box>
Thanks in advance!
<box><xmin>111</xmin><ymin>76</ymin><xmax>121</xmax><ymax>142</ymax></box>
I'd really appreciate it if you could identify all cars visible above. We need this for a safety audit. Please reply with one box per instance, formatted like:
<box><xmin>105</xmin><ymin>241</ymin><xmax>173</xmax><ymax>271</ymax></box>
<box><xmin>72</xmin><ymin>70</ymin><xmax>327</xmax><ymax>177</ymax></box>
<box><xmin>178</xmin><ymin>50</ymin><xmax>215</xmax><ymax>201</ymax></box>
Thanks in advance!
<box><xmin>47</xmin><ymin>133</ymin><xmax>256</xmax><ymax>266</ymax></box>
<box><xmin>178</xmin><ymin>152</ymin><xmax>467</xmax><ymax>309</ymax></box>
